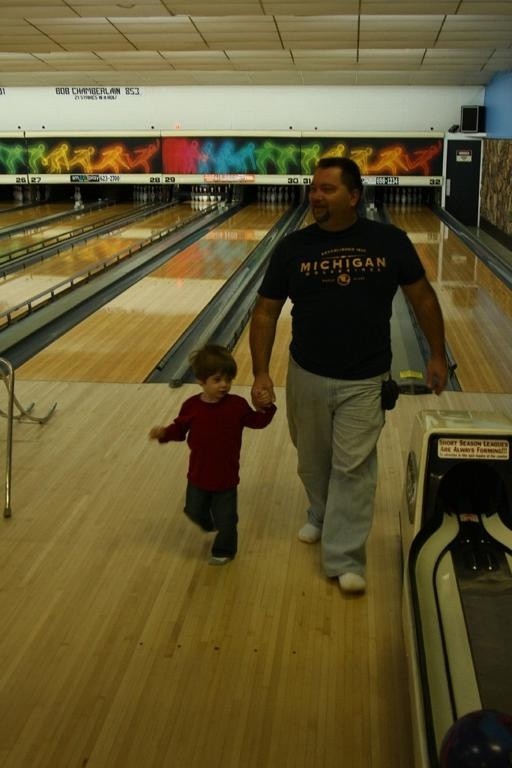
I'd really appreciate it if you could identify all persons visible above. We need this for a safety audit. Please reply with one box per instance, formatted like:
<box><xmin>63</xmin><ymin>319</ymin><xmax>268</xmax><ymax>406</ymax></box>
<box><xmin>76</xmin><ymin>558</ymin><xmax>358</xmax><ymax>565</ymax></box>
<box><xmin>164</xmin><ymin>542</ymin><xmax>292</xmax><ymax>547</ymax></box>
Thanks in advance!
<box><xmin>248</xmin><ymin>158</ymin><xmax>449</xmax><ymax>597</ymax></box>
<box><xmin>143</xmin><ymin>345</ymin><xmax>277</xmax><ymax>566</ymax></box>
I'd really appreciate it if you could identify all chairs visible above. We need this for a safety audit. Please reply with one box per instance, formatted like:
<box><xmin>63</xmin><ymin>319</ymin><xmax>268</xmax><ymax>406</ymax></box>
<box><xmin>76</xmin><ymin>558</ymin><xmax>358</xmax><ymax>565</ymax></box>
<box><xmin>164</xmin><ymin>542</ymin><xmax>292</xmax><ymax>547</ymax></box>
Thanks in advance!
<box><xmin>297</xmin><ymin>522</ymin><xmax>321</xmax><ymax>543</ymax></box>
<box><xmin>339</xmin><ymin>572</ymin><xmax>366</xmax><ymax>593</ymax></box>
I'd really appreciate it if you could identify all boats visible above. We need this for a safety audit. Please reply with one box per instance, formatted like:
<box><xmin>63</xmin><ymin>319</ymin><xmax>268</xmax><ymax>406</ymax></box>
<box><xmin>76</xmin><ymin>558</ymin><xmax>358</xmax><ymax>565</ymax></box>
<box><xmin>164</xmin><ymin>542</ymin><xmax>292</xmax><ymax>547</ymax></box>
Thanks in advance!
<box><xmin>461</xmin><ymin>104</ymin><xmax>486</xmax><ymax>132</ymax></box>
